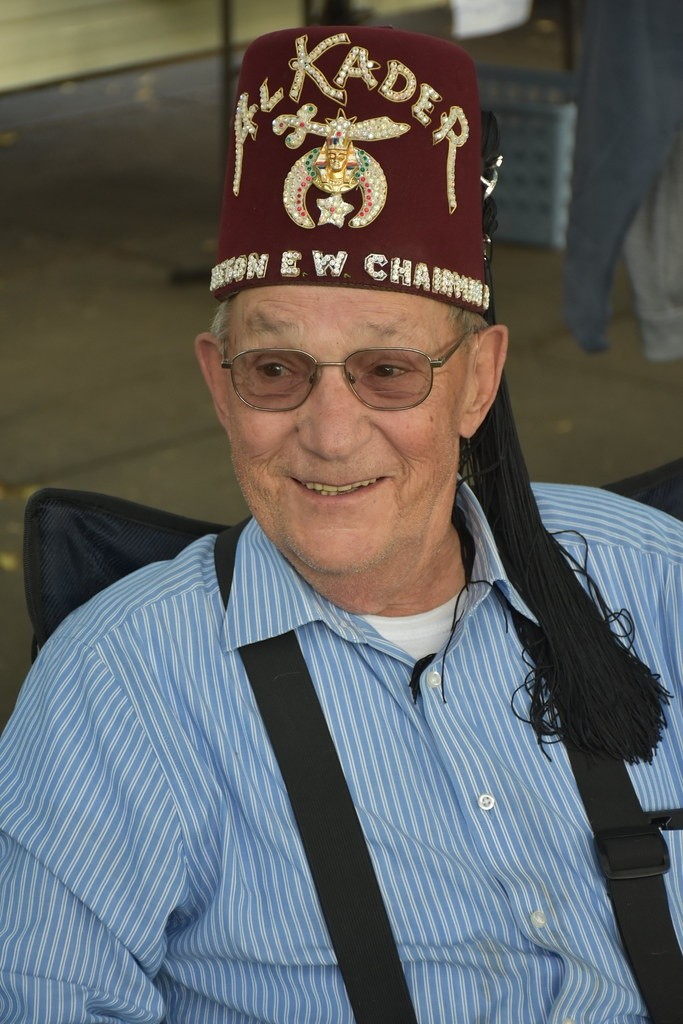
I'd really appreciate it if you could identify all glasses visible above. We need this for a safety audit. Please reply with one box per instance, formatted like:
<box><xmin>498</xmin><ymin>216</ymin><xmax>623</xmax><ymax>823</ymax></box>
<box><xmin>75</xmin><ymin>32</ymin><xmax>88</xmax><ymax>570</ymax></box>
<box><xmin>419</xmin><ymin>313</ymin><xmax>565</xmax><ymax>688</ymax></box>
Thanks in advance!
<box><xmin>221</xmin><ymin>328</ymin><xmax>473</xmax><ymax>413</ymax></box>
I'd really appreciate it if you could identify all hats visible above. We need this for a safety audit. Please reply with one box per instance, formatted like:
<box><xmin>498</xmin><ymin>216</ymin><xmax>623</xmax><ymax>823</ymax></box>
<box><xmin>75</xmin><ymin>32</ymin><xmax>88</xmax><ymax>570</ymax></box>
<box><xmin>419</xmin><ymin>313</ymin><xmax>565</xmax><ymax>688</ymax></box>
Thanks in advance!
<box><xmin>209</xmin><ymin>24</ymin><xmax>491</xmax><ymax>317</ymax></box>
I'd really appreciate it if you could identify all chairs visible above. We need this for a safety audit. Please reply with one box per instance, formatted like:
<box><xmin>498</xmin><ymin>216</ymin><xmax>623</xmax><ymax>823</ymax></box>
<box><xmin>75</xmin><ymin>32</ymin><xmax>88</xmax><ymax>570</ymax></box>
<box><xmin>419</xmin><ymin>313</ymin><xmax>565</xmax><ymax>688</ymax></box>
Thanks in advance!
<box><xmin>19</xmin><ymin>485</ymin><xmax>241</xmax><ymax>670</ymax></box>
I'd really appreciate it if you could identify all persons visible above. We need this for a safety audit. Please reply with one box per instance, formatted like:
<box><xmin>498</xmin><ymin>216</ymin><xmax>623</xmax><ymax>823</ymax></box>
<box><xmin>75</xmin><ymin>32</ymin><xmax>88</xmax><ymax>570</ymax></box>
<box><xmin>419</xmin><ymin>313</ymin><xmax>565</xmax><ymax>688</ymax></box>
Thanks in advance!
<box><xmin>0</xmin><ymin>26</ymin><xmax>680</xmax><ymax>1024</ymax></box>
